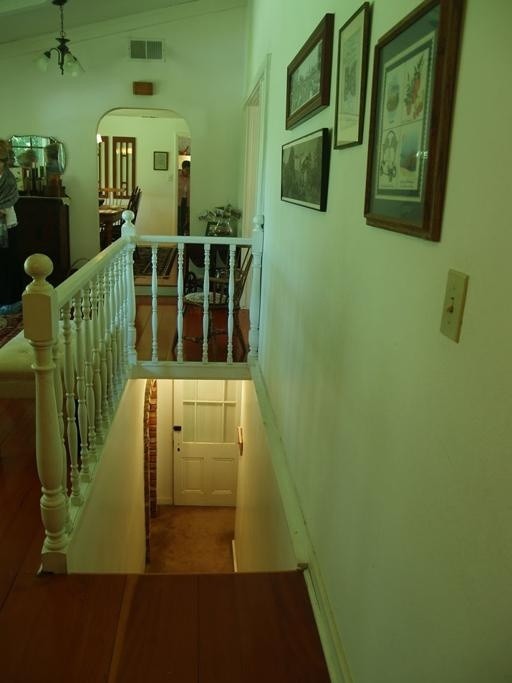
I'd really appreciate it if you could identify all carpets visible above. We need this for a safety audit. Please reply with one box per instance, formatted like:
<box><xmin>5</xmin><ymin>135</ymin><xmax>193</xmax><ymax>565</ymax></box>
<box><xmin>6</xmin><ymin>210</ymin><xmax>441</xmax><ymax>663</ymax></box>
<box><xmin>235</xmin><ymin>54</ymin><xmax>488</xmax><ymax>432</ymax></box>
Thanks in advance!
<box><xmin>0</xmin><ymin>310</ymin><xmax>25</xmax><ymax>348</ymax></box>
<box><xmin>126</xmin><ymin>247</ymin><xmax>177</xmax><ymax>280</ymax></box>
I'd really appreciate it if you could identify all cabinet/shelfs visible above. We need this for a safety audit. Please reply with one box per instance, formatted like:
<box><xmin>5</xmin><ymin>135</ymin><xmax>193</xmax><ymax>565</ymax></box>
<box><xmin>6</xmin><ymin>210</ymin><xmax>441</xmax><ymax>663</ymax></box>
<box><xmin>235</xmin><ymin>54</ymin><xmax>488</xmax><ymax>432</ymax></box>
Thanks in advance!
<box><xmin>183</xmin><ymin>233</ymin><xmax>240</xmax><ymax>310</ymax></box>
<box><xmin>15</xmin><ymin>196</ymin><xmax>69</xmax><ymax>283</ymax></box>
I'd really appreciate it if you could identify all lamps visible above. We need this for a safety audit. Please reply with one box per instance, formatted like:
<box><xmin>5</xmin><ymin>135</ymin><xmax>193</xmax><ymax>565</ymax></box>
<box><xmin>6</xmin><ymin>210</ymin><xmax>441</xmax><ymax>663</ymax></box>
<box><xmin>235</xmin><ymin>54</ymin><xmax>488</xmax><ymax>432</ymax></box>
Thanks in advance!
<box><xmin>36</xmin><ymin>0</ymin><xmax>85</xmax><ymax>77</ymax></box>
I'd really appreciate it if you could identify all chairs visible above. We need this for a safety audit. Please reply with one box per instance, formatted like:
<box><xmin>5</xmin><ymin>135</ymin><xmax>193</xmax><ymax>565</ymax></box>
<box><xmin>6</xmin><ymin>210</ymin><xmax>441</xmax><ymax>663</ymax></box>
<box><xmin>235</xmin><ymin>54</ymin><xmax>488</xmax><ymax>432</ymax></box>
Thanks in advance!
<box><xmin>170</xmin><ymin>244</ymin><xmax>254</xmax><ymax>362</ymax></box>
<box><xmin>111</xmin><ymin>186</ymin><xmax>141</xmax><ymax>262</ymax></box>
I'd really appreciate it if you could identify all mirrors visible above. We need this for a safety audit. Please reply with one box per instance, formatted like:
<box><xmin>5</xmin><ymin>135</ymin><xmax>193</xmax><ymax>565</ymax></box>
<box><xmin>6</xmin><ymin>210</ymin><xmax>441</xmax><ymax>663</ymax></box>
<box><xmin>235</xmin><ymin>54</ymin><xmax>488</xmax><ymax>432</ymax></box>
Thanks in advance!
<box><xmin>2</xmin><ymin>134</ymin><xmax>66</xmax><ymax>181</ymax></box>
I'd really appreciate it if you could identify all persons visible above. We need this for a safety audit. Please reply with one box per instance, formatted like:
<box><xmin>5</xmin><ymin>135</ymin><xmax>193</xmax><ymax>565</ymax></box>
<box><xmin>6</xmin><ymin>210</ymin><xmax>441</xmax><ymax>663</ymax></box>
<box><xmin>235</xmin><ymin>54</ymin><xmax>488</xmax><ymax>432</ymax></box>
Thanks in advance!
<box><xmin>0</xmin><ymin>138</ymin><xmax>21</xmax><ymax>313</ymax></box>
<box><xmin>178</xmin><ymin>160</ymin><xmax>190</xmax><ymax>236</ymax></box>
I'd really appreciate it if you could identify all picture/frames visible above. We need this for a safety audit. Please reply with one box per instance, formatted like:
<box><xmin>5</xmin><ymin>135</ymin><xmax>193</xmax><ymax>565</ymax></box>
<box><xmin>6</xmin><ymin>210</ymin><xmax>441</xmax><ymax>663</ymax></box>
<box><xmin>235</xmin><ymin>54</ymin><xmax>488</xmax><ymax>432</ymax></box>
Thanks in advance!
<box><xmin>153</xmin><ymin>151</ymin><xmax>168</xmax><ymax>170</ymax></box>
<box><xmin>281</xmin><ymin>129</ymin><xmax>328</xmax><ymax>210</ymax></box>
<box><xmin>286</xmin><ymin>12</ymin><xmax>334</xmax><ymax>130</ymax></box>
<box><xmin>363</xmin><ymin>0</ymin><xmax>463</xmax><ymax>241</ymax></box>
<box><xmin>334</xmin><ymin>3</ymin><xmax>370</xmax><ymax>149</ymax></box>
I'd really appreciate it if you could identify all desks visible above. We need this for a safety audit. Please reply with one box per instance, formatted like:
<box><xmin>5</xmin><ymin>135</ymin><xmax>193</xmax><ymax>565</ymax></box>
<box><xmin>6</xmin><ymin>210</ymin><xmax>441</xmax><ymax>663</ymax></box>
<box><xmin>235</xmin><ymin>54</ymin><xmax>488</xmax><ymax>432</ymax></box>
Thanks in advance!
<box><xmin>99</xmin><ymin>206</ymin><xmax>125</xmax><ymax>248</ymax></box>
